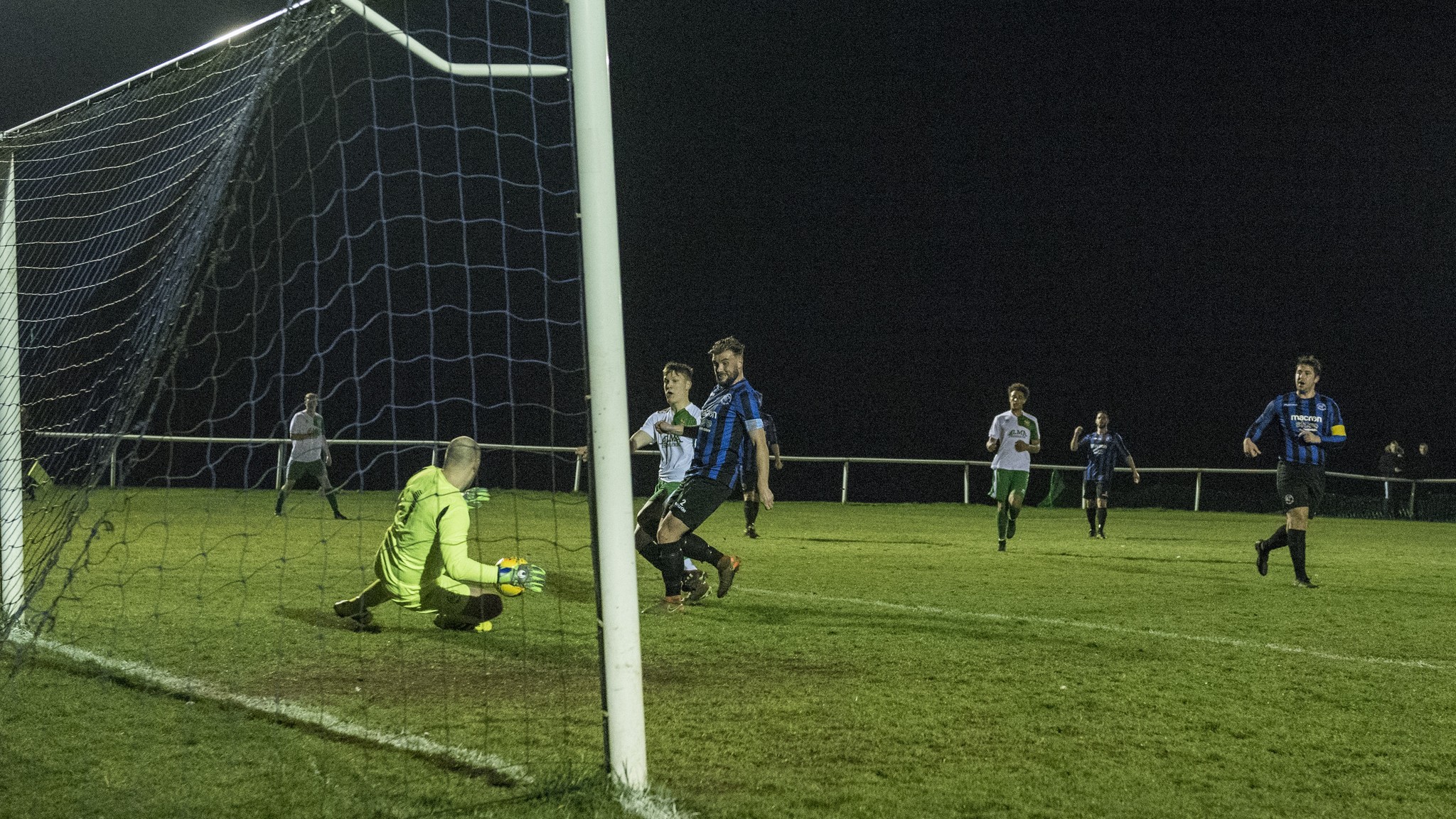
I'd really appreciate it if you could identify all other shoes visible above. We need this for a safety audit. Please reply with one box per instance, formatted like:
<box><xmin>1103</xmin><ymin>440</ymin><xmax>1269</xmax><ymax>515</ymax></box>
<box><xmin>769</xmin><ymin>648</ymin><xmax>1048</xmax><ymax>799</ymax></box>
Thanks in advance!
<box><xmin>744</xmin><ymin>527</ymin><xmax>760</xmax><ymax>539</ymax></box>
<box><xmin>1089</xmin><ymin>530</ymin><xmax>1096</xmax><ymax>536</ymax></box>
<box><xmin>335</xmin><ymin>514</ymin><xmax>347</xmax><ymax>520</ymax></box>
<box><xmin>274</xmin><ymin>511</ymin><xmax>281</xmax><ymax>516</ymax></box>
<box><xmin>1097</xmin><ymin>533</ymin><xmax>1107</xmax><ymax>539</ymax></box>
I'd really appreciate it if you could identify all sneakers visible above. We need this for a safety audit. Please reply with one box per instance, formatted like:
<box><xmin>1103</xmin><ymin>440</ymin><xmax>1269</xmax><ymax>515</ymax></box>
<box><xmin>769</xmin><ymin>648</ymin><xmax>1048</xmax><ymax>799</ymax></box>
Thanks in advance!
<box><xmin>717</xmin><ymin>555</ymin><xmax>742</xmax><ymax>599</ymax></box>
<box><xmin>681</xmin><ymin>583</ymin><xmax>713</xmax><ymax>605</ymax></box>
<box><xmin>334</xmin><ymin>599</ymin><xmax>374</xmax><ymax>624</ymax></box>
<box><xmin>1007</xmin><ymin>520</ymin><xmax>1016</xmax><ymax>539</ymax></box>
<box><xmin>1254</xmin><ymin>539</ymin><xmax>1269</xmax><ymax>576</ymax></box>
<box><xmin>998</xmin><ymin>540</ymin><xmax>1006</xmax><ymax>551</ymax></box>
<box><xmin>644</xmin><ymin>599</ymin><xmax>687</xmax><ymax>616</ymax></box>
<box><xmin>1293</xmin><ymin>580</ymin><xmax>1316</xmax><ymax>589</ymax></box>
<box><xmin>681</xmin><ymin>570</ymin><xmax>706</xmax><ymax>591</ymax></box>
<box><xmin>434</xmin><ymin>613</ymin><xmax>492</xmax><ymax>634</ymax></box>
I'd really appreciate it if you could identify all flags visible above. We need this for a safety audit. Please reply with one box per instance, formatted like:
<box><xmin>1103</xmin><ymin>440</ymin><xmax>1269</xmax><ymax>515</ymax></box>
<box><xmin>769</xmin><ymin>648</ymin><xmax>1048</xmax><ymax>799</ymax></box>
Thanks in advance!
<box><xmin>28</xmin><ymin>460</ymin><xmax>52</xmax><ymax>491</ymax></box>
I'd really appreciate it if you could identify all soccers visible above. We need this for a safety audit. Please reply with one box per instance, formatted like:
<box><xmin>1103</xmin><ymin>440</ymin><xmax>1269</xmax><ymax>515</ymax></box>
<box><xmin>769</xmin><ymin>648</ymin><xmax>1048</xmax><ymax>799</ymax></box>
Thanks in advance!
<box><xmin>493</xmin><ymin>555</ymin><xmax>529</xmax><ymax>597</ymax></box>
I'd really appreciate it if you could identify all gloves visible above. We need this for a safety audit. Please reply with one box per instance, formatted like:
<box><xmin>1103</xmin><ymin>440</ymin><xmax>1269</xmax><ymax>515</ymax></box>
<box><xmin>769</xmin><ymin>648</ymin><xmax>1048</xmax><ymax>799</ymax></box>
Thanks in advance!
<box><xmin>498</xmin><ymin>563</ymin><xmax>546</xmax><ymax>593</ymax></box>
<box><xmin>464</xmin><ymin>488</ymin><xmax>490</xmax><ymax>509</ymax></box>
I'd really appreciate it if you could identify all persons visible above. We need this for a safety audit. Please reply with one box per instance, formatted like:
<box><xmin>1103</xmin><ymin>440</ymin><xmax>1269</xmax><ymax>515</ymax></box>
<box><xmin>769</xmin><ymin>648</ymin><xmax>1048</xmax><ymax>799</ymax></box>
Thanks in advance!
<box><xmin>1070</xmin><ymin>411</ymin><xmax>1139</xmax><ymax>539</ymax></box>
<box><xmin>274</xmin><ymin>393</ymin><xmax>348</xmax><ymax>520</ymax></box>
<box><xmin>986</xmin><ymin>383</ymin><xmax>1040</xmax><ymax>552</ymax></box>
<box><xmin>20</xmin><ymin>406</ymin><xmax>36</xmax><ymax>500</ymax></box>
<box><xmin>739</xmin><ymin>391</ymin><xmax>783</xmax><ymax>538</ymax></box>
<box><xmin>575</xmin><ymin>362</ymin><xmax>713</xmax><ymax>603</ymax></box>
<box><xmin>1243</xmin><ymin>356</ymin><xmax>1347</xmax><ymax>588</ymax></box>
<box><xmin>334</xmin><ymin>436</ymin><xmax>546</xmax><ymax>632</ymax></box>
<box><xmin>643</xmin><ymin>337</ymin><xmax>773</xmax><ymax>615</ymax></box>
<box><xmin>1378</xmin><ymin>441</ymin><xmax>1435</xmax><ymax>521</ymax></box>
